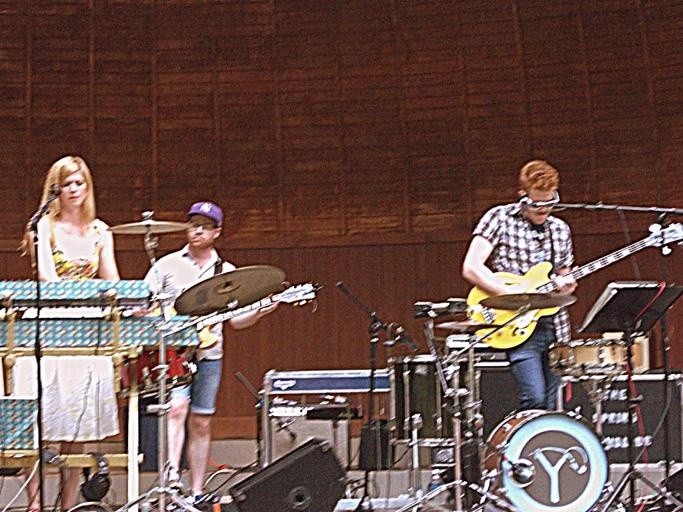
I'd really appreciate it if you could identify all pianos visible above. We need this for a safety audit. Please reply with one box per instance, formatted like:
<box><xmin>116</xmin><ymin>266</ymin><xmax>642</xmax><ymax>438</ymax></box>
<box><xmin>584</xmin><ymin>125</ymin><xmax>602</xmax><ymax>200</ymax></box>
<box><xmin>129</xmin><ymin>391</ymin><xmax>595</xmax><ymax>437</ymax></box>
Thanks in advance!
<box><xmin>0</xmin><ymin>315</ymin><xmax>202</xmax><ymax>357</ymax></box>
<box><xmin>0</xmin><ymin>279</ymin><xmax>152</xmax><ymax>310</ymax></box>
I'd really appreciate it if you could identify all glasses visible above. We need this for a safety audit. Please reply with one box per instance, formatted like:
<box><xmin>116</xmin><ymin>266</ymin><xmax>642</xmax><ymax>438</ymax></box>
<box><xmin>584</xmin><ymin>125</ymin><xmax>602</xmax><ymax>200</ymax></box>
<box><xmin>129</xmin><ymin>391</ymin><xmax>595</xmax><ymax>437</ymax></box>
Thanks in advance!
<box><xmin>521</xmin><ymin>190</ymin><xmax>560</xmax><ymax>210</ymax></box>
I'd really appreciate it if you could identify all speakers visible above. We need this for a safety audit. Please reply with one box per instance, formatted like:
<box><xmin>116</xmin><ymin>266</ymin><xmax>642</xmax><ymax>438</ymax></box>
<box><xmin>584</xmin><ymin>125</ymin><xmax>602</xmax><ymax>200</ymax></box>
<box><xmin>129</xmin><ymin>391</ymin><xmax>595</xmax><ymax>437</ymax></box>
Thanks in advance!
<box><xmin>229</xmin><ymin>436</ymin><xmax>348</xmax><ymax>512</ymax></box>
<box><xmin>556</xmin><ymin>374</ymin><xmax>682</xmax><ymax>472</ymax></box>
<box><xmin>360</xmin><ymin>419</ymin><xmax>395</xmax><ymax>470</ymax></box>
<box><xmin>396</xmin><ymin>362</ymin><xmax>521</xmax><ymax>438</ymax></box>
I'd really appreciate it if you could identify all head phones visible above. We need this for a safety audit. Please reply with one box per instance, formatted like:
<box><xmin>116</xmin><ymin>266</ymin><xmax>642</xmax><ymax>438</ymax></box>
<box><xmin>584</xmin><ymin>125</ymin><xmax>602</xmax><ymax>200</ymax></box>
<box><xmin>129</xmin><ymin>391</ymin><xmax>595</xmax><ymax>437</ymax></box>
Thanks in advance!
<box><xmin>80</xmin><ymin>451</ymin><xmax>111</xmax><ymax>501</ymax></box>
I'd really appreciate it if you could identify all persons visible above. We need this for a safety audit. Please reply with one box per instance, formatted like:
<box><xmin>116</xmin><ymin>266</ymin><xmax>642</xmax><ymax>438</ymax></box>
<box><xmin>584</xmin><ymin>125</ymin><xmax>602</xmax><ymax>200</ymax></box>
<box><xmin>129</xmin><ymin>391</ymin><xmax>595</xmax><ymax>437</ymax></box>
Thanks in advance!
<box><xmin>8</xmin><ymin>156</ymin><xmax>121</xmax><ymax>512</ymax></box>
<box><xmin>462</xmin><ymin>159</ymin><xmax>577</xmax><ymax>410</ymax></box>
<box><xmin>133</xmin><ymin>201</ymin><xmax>280</xmax><ymax>500</ymax></box>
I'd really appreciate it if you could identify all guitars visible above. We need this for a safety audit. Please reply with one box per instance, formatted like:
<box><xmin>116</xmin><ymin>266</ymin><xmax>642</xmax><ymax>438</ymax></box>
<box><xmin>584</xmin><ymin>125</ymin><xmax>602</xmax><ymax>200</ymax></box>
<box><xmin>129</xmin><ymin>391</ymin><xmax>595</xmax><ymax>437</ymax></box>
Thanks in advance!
<box><xmin>142</xmin><ymin>284</ymin><xmax>317</xmax><ymax>349</ymax></box>
<box><xmin>465</xmin><ymin>220</ymin><xmax>683</xmax><ymax>351</ymax></box>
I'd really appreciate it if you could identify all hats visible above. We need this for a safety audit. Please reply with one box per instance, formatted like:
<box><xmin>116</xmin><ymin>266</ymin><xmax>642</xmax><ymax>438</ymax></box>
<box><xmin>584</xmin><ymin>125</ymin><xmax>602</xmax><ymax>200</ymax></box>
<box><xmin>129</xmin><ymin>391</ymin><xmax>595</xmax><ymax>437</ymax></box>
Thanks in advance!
<box><xmin>187</xmin><ymin>201</ymin><xmax>223</xmax><ymax>226</ymax></box>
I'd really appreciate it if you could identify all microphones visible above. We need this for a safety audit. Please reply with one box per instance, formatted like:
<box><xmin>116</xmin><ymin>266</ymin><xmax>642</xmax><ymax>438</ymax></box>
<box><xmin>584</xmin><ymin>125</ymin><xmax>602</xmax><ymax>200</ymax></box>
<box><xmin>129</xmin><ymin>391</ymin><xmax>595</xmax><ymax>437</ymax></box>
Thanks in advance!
<box><xmin>390</xmin><ymin>320</ymin><xmax>422</xmax><ymax>353</ymax></box>
<box><xmin>31</xmin><ymin>183</ymin><xmax>63</xmax><ymax>229</ymax></box>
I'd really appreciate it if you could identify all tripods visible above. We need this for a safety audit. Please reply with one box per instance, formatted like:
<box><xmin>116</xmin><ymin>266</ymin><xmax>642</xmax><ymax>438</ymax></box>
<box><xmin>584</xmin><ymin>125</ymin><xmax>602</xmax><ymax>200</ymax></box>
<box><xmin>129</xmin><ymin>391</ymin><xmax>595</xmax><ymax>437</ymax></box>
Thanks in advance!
<box><xmin>115</xmin><ymin>326</ymin><xmax>204</xmax><ymax>512</ymax></box>
<box><xmin>601</xmin><ymin>330</ymin><xmax>683</xmax><ymax>512</ymax></box>
<box><xmin>394</xmin><ymin>362</ymin><xmax>519</xmax><ymax>512</ymax></box>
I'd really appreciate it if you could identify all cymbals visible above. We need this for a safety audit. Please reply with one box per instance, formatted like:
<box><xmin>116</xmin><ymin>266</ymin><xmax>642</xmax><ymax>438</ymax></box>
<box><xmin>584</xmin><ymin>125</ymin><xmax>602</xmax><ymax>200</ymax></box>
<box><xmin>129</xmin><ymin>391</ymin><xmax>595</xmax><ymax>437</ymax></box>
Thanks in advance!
<box><xmin>172</xmin><ymin>264</ymin><xmax>286</xmax><ymax>317</ymax></box>
<box><xmin>107</xmin><ymin>221</ymin><xmax>195</xmax><ymax>237</ymax></box>
<box><xmin>435</xmin><ymin>321</ymin><xmax>504</xmax><ymax>334</ymax></box>
<box><xmin>477</xmin><ymin>290</ymin><xmax>577</xmax><ymax>311</ymax></box>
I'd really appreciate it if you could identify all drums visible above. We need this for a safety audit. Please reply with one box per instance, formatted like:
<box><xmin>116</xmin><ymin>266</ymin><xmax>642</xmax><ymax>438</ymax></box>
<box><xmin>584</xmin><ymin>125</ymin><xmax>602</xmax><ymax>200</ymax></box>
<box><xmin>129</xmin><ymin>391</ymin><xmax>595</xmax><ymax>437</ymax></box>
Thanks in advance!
<box><xmin>118</xmin><ymin>356</ymin><xmax>150</xmax><ymax>397</ymax></box>
<box><xmin>139</xmin><ymin>345</ymin><xmax>193</xmax><ymax>395</ymax></box>
<box><xmin>547</xmin><ymin>336</ymin><xmax>651</xmax><ymax>380</ymax></box>
<box><xmin>479</xmin><ymin>408</ymin><xmax>609</xmax><ymax>512</ymax></box>
<box><xmin>387</xmin><ymin>355</ymin><xmax>481</xmax><ymax>447</ymax></box>
